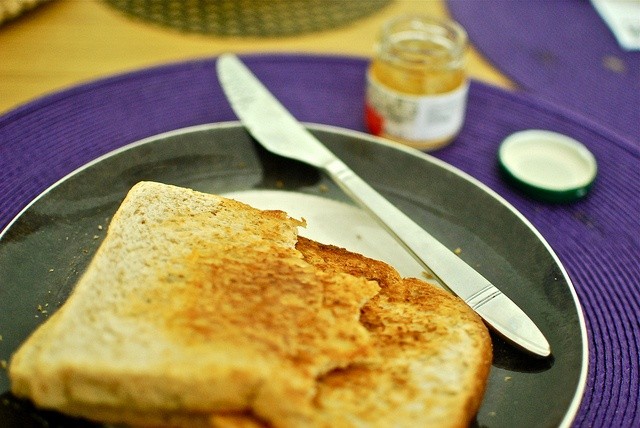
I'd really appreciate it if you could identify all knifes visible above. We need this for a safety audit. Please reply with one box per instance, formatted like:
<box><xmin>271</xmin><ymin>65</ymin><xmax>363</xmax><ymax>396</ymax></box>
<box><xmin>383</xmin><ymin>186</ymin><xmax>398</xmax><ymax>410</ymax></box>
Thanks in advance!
<box><xmin>215</xmin><ymin>52</ymin><xmax>552</xmax><ymax>362</ymax></box>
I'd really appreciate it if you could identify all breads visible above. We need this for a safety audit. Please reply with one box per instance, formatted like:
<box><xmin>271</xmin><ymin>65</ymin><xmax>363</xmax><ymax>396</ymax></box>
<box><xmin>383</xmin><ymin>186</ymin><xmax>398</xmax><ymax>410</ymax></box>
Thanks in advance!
<box><xmin>8</xmin><ymin>180</ymin><xmax>380</xmax><ymax>416</ymax></box>
<box><xmin>92</xmin><ymin>233</ymin><xmax>496</xmax><ymax>428</ymax></box>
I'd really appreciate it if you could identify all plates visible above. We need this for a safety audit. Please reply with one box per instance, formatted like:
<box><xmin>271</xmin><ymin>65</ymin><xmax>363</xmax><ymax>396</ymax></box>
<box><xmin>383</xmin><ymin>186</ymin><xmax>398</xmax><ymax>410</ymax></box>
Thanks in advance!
<box><xmin>0</xmin><ymin>120</ymin><xmax>591</xmax><ymax>427</ymax></box>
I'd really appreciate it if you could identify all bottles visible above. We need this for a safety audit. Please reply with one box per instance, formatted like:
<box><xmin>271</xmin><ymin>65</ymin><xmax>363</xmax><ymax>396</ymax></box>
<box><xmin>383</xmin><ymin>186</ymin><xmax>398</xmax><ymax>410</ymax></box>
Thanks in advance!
<box><xmin>367</xmin><ymin>15</ymin><xmax>474</xmax><ymax>149</ymax></box>
